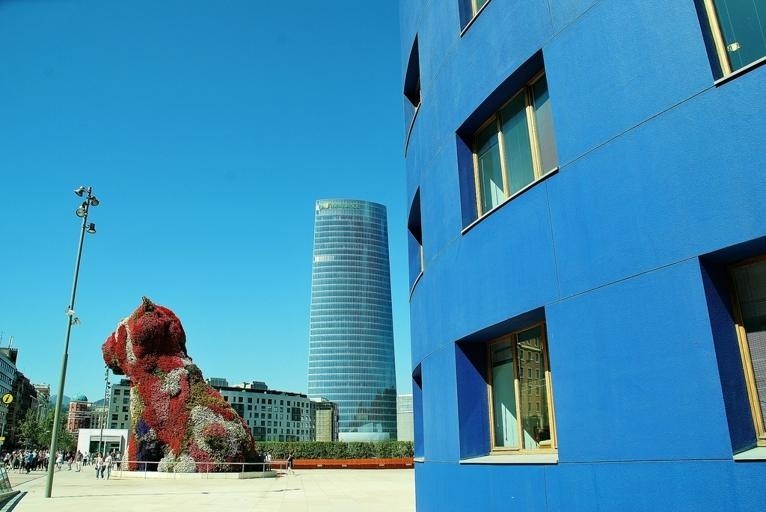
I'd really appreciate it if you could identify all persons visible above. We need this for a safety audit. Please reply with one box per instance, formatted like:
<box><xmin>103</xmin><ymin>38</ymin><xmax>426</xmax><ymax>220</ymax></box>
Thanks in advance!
<box><xmin>2</xmin><ymin>449</ymin><xmax>122</xmax><ymax>480</ymax></box>
<box><xmin>287</xmin><ymin>453</ymin><xmax>296</xmax><ymax>475</ymax></box>
<box><xmin>266</xmin><ymin>452</ymin><xmax>272</xmax><ymax>471</ymax></box>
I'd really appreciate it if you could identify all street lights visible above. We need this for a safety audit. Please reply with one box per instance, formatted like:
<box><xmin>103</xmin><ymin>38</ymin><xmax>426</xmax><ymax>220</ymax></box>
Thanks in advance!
<box><xmin>38</xmin><ymin>183</ymin><xmax>102</xmax><ymax>499</ymax></box>
